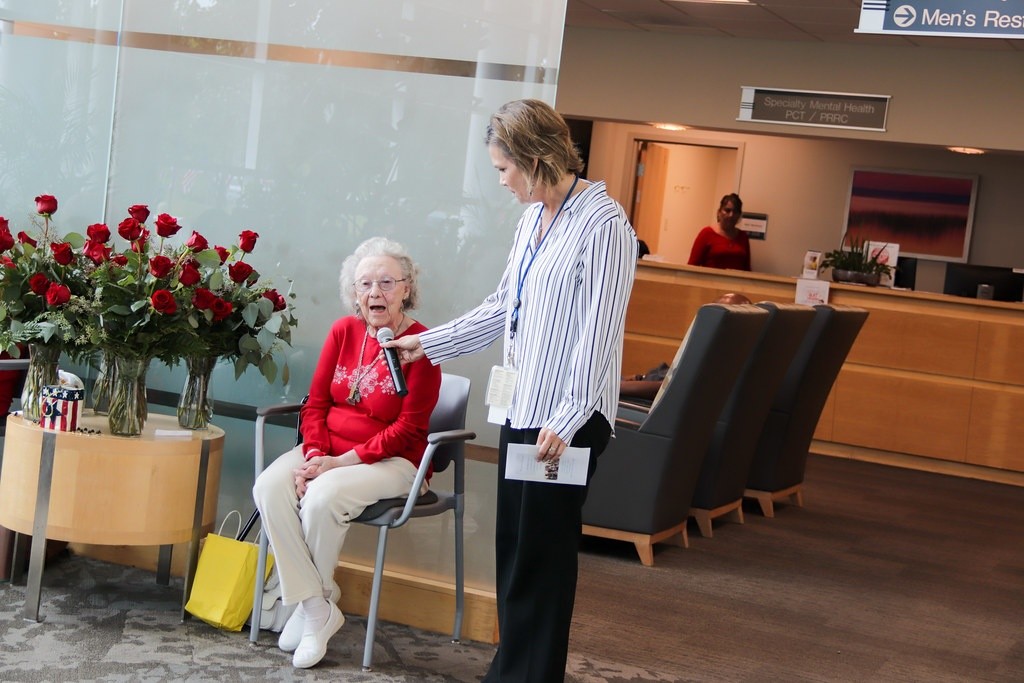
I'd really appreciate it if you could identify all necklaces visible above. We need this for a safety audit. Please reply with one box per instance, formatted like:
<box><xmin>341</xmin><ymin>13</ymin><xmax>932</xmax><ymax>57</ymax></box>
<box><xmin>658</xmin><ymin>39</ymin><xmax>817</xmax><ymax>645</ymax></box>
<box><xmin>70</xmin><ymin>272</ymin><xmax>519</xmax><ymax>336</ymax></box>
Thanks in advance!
<box><xmin>345</xmin><ymin>312</ymin><xmax>405</xmax><ymax>406</ymax></box>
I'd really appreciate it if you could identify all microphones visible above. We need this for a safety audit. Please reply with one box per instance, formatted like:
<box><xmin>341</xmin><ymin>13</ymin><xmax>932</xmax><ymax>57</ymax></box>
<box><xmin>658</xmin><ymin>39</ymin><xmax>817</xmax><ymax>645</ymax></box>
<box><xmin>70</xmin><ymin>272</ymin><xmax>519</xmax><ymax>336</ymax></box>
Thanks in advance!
<box><xmin>377</xmin><ymin>327</ymin><xmax>407</xmax><ymax>397</ymax></box>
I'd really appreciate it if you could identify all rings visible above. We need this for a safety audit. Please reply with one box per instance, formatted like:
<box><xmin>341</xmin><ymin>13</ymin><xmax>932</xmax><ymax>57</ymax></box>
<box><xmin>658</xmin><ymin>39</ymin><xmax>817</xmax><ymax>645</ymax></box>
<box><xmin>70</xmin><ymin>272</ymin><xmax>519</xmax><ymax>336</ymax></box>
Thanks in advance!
<box><xmin>549</xmin><ymin>446</ymin><xmax>557</xmax><ymax>451</ymax></box>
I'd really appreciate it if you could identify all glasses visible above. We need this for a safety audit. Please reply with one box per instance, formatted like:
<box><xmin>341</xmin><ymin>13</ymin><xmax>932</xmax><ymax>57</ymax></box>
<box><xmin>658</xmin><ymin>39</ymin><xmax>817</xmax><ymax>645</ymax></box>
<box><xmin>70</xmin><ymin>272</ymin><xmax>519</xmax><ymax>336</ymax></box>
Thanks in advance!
<box><xmin>352</xmin><ymin>276</ymin><xmax>407</xmax><ymax>292</ymax></box>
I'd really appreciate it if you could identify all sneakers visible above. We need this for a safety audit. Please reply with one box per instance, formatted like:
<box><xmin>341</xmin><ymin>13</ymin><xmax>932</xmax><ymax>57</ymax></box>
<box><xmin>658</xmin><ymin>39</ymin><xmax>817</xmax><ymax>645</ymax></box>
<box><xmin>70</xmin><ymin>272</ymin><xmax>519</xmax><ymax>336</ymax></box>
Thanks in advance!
<box><xmin>293</xmin><ymin>597</ymin><xmax>345</xmax><ymax>668</ymax></box>
<box><xmin>278</xmin><ymin>580</ymin><xmax>342</xmax><ymax>652</ymax></box>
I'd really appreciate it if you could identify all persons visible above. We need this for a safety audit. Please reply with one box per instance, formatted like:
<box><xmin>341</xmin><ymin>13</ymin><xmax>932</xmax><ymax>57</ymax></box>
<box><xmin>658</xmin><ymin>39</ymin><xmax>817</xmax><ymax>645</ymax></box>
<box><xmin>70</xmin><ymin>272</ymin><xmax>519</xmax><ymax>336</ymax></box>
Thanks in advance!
<box><xmin>687</xmin><ymin>192</ymin><xmax>751</xmax><ymax>271</ymax></box>
<box><xmin>252</xmin><ymin>238</ymin><xmax>442</xmax><ymax>670</ymax></box>
<box><xmin>379</xmin><ymin>100</ymin><xmax>638</xmax><ymax>683</ymax></box>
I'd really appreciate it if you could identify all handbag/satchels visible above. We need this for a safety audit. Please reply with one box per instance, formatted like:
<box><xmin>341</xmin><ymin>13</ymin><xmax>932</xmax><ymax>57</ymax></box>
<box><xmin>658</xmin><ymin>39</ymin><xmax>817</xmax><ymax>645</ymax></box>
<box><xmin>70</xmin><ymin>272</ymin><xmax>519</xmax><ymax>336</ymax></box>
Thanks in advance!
<box><xmin>185</xmin><ymin>510</ymin><xmax>274</xmax><ymax>632</ymax></box>
<box><xmin>245</xmin><ymin>544</ymin><xmax>298</xmax><ymax>632</ymax></box>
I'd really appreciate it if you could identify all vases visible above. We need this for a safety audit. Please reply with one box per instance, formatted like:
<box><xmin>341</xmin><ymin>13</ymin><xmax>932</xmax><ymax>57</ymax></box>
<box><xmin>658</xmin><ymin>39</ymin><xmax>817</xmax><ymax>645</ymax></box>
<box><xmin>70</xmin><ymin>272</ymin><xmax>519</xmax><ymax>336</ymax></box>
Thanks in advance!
<box><xmin>20</xmin><ymin>344</ymin><xmax>215</xmax><ymax>437</ymax></box>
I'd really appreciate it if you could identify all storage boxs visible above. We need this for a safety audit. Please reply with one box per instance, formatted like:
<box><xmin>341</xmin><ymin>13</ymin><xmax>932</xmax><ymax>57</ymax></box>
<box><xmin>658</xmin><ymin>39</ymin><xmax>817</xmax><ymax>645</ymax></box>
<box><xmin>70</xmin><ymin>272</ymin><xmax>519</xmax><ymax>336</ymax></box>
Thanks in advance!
<box><xmin>40</xmin><ymin>386</ymin><xmax>85</xmax><ymax>432</ymax></box>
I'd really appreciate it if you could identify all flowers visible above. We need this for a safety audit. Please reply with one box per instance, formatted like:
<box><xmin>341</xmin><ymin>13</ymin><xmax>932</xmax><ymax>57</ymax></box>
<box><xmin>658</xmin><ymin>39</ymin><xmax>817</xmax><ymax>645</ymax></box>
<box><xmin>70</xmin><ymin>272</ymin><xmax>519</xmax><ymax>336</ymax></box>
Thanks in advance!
<box><xmin>0</xmin><ymin>192</ymin><xmax>298</xmax><ymax>388</ymax></box>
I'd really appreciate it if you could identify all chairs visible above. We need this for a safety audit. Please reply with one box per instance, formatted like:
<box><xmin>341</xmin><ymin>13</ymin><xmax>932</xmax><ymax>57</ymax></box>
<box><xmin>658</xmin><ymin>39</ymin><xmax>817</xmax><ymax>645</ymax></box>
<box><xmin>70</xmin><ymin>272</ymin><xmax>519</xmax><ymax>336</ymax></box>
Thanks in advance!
<box><xmin>247</xmin><ymin>371</ymin><xmax>476</xmax><ymax>672</ymax></box>
<box><xmin>582</xmin><ymin>300</ymin><xmax>872</xmax><ymax>567</ymax></box>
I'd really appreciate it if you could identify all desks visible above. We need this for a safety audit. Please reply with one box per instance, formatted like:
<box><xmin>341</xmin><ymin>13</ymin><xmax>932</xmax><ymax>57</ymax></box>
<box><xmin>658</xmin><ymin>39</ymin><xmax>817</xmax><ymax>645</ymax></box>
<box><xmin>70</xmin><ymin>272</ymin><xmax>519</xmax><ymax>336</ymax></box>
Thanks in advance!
<box><xmin>0</xmin><ymin>405</ymin><xmax>226</xmax><ymax>624</ymax></box>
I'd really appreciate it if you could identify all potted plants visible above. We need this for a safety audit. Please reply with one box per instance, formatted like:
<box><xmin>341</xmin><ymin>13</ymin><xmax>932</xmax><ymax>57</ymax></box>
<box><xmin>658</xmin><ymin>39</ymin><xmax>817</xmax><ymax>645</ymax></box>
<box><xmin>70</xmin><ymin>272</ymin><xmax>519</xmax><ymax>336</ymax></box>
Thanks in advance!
<box><xmin>818</xmin><ymin>227</ymin><xmax>899</xmax><ymax>286</ymax></box>
